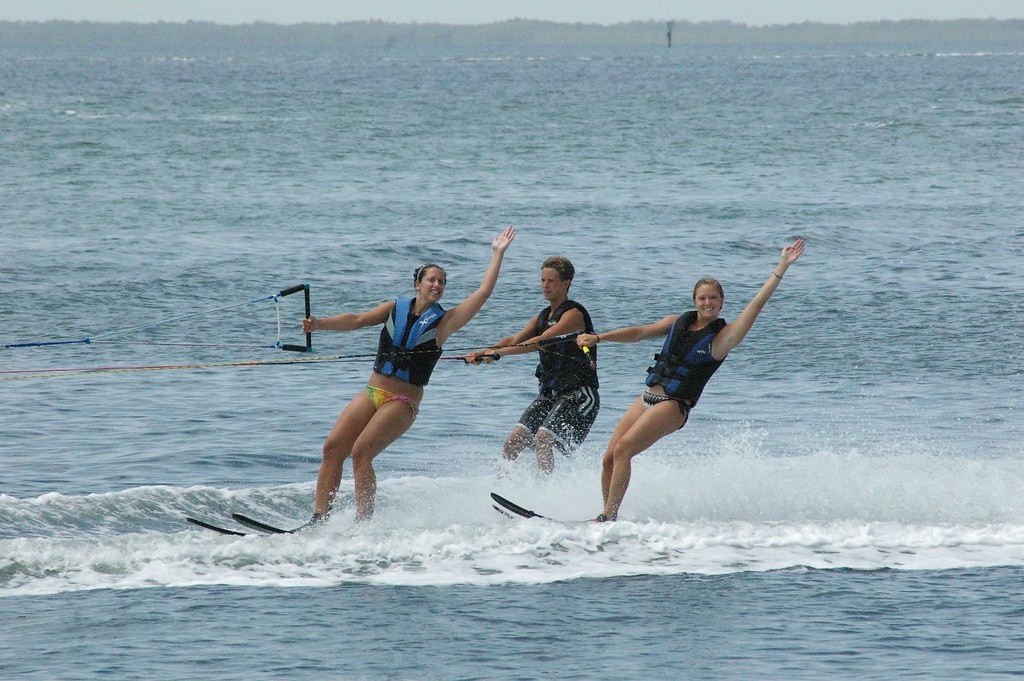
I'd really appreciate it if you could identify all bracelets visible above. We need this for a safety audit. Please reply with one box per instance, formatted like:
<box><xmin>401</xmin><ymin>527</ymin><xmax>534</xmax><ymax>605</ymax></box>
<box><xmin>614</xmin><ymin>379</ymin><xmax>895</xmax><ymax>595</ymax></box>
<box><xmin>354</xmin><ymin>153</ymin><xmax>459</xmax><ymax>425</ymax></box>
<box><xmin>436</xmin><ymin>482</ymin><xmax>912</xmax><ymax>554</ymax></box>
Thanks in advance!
<box><xmin>773</xmin><ymin>272</ymin><xmax>783</xmax><ymax>279</ymax></box>
<box><xmin>596</xmin><ymin>334</ymin><xmax>600</xmax><ymax>343</ymax></box>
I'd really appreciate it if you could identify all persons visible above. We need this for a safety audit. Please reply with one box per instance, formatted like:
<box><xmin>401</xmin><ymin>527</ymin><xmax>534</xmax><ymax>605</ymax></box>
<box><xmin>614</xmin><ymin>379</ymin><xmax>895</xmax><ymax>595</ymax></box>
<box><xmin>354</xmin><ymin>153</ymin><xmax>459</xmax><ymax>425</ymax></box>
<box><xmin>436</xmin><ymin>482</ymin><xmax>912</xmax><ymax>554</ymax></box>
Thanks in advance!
<box><xmin>575</xmin><ymin>237</ymin><xmax>805</xmax><ymax>523</ymax></box>
<box><xmin>463</xmin><ymin>254</ymin><xmax>600</xmax><ymax>484</ymax></box>
<box><xmin>301</xmin><ymin>223</ymin><xmax>516</xmax><ymax>530</ymax></box>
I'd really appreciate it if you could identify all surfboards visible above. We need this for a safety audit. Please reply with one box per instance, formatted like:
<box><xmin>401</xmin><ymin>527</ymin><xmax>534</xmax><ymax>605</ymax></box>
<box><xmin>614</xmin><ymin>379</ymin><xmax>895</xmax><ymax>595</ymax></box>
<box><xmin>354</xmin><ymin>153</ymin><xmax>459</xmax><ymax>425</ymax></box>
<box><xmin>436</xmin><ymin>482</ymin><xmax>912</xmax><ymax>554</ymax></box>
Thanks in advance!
<box><xmin>488</xmin><ymin>488</ymin><xmax>565</xmax><ymax>522</ymax></box>
<box><xmin>182</xmin><ymin>511</ymin><xmax>299</xmax><ymax>536</ymax></box>
